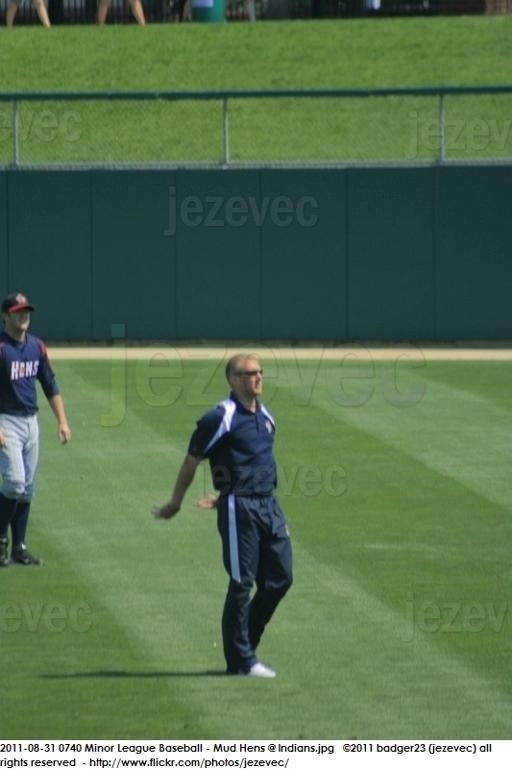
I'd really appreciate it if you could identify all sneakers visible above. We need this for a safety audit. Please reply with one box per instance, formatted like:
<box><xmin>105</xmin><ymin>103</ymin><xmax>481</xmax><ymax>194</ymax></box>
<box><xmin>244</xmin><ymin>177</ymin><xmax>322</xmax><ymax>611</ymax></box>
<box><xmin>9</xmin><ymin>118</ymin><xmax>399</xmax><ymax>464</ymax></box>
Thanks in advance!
<box><xmin>227</xmin><ymin>660</ymin><xmax>277</xmax><ymax>679</ymax></box>
<box><xmin>10</xmin><ymin>543</ymin><xmax>42</xmax><ymax>567</ymax></box>
<box><xmin>0</xmin><ymin>537</ymin><xmax>12</xmax><ymax>567</ymax></box>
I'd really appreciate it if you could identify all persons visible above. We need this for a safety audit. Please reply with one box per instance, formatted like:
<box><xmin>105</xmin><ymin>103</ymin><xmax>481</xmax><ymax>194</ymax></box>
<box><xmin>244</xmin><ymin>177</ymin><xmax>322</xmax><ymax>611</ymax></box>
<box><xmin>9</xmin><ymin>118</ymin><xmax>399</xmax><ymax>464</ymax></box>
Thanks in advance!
<box><xmin>150</xmin><ymin>351</ymin><xmax>295</xmax><ymax>678</ymax></box>
<box><xmin>2</xmin><ymin>0</ymin><xmax>258</xmax><ymax>27</ymax></box>
<box><xmin>1</xmin><ymin>290</ymin><xmax>71</xmax><ymax>568</ymax></box>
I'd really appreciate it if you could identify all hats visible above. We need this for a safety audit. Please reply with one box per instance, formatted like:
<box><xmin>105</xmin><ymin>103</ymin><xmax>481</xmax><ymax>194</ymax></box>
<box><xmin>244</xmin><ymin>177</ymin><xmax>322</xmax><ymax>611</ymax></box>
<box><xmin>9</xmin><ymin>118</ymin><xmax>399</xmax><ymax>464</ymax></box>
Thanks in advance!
<box><xmin>0</xmin><ymin>292</ymin><xmax>35</xmax><ymax>313</ymax></box>
